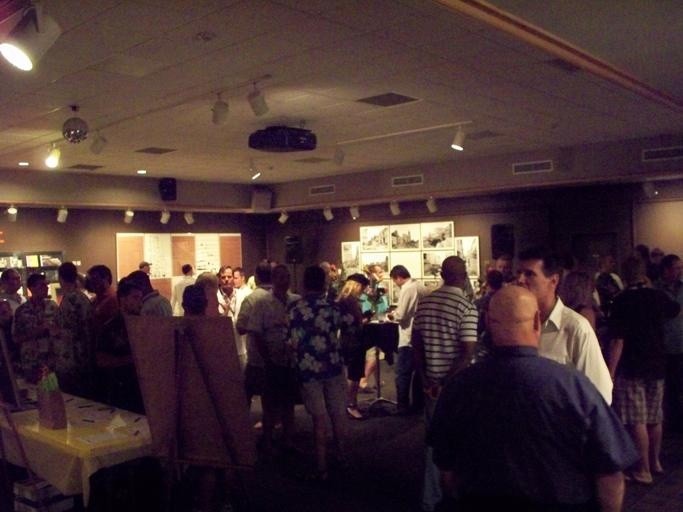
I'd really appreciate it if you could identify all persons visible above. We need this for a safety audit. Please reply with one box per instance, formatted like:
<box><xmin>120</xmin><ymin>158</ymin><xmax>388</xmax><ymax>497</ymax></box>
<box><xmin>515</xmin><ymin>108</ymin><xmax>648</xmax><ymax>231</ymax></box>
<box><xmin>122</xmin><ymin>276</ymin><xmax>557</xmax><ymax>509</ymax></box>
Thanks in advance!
<box><xmin>0</xmin><ymin>235</ymin><xmax>682</xmax><ymax>512</ymax></box>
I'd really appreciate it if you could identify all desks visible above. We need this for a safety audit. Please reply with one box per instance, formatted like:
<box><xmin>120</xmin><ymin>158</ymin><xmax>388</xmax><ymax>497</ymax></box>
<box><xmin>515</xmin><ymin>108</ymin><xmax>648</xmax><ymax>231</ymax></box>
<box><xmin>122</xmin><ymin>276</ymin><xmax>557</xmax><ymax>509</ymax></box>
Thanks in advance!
<box><xmin>349</xmin><ymin>313</ymin><xmax>402</xmax><ymax>418</ymax></box>
<box><xmin>2</xmin><ymin>379</ymin><xmax>156</xmax><ymax>510</ymax></box>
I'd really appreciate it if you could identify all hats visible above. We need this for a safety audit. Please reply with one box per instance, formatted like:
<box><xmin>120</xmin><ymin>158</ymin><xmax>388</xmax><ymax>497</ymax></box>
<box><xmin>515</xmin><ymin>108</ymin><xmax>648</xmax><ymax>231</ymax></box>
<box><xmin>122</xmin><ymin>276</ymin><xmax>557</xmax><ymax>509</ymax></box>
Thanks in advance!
<box><xmin>347</xmin><ymin>273</ymin><xmax>368</xmax><ymax>287</ymax></box>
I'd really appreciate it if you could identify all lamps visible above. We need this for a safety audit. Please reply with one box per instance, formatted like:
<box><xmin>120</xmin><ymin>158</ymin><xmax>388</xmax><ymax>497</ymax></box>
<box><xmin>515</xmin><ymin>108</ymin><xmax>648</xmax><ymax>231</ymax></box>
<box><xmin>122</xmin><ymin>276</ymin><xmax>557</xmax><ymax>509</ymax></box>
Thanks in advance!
<box><xmin>450</xmin><ymin>125</ymin><xmax>471</xmax><ymax>155</ymax></box>
<box><xmin>62</xmin><ymin>115</ymin><xmax>91</xmax><ymax>147</ymax></box>
<box><xmin>0</xmin><ymin>1</ymin><xmax>68</xmax><ymax>73</ymax></box>
<box><xmin>274</xmin><ymin>195</ymin><xmax>442</xmax><ymax>223</ymax></box>
<box><xmin>45</xmin><ymin>76</ymin><xmax>272</xmax><ymax>171</ymax></box>
<box><xmin>3</xmin><ymin>199</ymin><xmax>200</xmax><ymax>227</ymax></box>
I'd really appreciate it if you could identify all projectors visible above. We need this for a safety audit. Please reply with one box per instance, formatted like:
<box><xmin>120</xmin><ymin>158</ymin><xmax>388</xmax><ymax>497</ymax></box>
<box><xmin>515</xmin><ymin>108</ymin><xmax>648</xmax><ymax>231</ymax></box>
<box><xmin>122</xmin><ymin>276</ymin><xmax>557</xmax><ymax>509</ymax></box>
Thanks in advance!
<box><xmin>247</xmin><ymin>126</ymin><xmax>316</xmax><ymax>151</ymax></box>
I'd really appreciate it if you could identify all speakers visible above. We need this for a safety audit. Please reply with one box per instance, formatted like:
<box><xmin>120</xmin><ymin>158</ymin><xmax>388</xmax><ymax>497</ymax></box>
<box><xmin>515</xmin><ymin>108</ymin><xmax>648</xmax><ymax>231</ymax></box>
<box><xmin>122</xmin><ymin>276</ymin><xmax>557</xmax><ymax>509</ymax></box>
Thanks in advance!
<box><xmin>159</xmin><ymin>178</ymin><xmax>177</xmax><ymax>202</ymax></box>
<box><xmin>492</xmin><ymin>224</ymin><xmax>515</xmax><ymax>259</ymax></box>
<box><xmin>286</xmin><ymin>248</ymin><xmax>303</xmax><ymax>263</ymax></box>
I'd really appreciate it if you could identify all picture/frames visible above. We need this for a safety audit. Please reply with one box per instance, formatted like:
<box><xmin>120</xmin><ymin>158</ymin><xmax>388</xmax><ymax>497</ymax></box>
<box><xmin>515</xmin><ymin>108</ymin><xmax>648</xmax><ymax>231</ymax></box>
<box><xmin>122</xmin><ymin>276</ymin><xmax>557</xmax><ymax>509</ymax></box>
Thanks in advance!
<box><xmin>338</xmin><ymin>220</ymin><xmax>484</xmax><ymax>307</ymax></box>
